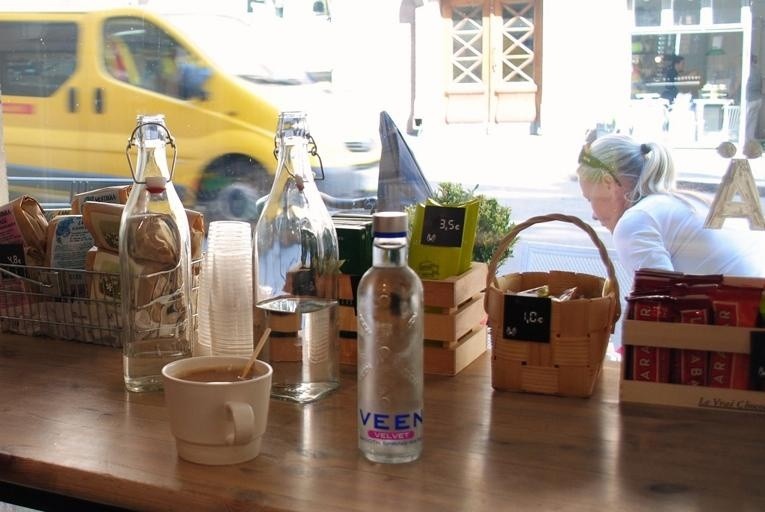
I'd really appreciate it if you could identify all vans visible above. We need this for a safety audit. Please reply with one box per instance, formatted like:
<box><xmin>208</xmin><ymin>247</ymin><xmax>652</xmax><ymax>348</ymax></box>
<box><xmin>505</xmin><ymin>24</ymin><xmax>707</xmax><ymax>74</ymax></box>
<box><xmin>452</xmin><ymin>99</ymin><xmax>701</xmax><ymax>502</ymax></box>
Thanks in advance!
<box><xmin>0</xmin><ymin>7</ymin><xmax>325</xmax><ymax>237</ymax></box>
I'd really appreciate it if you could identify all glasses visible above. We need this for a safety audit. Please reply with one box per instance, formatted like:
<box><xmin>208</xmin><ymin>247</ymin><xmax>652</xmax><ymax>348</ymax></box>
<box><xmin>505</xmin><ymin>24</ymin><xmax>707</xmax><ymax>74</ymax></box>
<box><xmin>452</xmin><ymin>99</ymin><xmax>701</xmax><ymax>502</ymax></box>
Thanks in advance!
<box><xmin>578</xmin><ymin>142</ymin><xmax>621</xmax><ymax>187</ymax></box>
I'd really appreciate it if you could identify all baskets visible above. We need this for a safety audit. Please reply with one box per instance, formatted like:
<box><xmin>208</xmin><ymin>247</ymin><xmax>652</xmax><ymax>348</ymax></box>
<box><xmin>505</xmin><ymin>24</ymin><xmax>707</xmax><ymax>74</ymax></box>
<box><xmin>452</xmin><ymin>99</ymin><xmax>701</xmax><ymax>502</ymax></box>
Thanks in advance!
<box><xmin>480</xmin><ymin>210</ymin><xmax>623</xmax><ymax>400</ymax></box>
<box><xmin>1</xmin><ymin>254</ymin><xmax>215</xmax><ymax>350</ymax></box>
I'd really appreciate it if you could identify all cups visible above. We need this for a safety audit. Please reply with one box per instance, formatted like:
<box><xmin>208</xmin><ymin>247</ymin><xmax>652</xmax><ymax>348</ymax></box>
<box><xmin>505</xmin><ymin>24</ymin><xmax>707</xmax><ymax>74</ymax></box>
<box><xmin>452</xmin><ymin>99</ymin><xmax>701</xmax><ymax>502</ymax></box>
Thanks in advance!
<box><xmin>195</xmin><ymin>216</ymin><xmax>256</xmax><ymax>354</ymax></box>
<box><xmin>163</xmin><ymin>354</ymin><xmax>273</xmax><ymax>467</ymax></box>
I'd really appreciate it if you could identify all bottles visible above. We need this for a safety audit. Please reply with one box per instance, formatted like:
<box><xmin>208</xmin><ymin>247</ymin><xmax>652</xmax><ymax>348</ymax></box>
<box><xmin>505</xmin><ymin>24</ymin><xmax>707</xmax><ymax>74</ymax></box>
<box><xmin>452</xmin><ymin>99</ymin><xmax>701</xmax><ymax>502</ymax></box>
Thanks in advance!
<box><xmin>118</xmin><ymin>112</ymin><xmax>193</xmax><ymax>395</ymax></box>
<box><xmin>353</xmin><ymin>208</ymin><xmax>427</xmax><ymax>467</ymax></box>
<box><xmin>250</xmin><ymin>111</ymin><xmax>345</xmax><ymax>405</ymax></box>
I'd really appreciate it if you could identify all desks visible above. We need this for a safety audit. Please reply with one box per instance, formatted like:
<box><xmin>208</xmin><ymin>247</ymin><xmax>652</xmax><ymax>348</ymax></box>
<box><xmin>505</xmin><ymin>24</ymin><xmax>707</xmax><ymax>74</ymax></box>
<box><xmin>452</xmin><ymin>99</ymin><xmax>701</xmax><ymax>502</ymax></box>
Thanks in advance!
<box><xmin>1</xmin><ymin>332</ymin><xmax>765</xmax><ymax>509</ymax></box>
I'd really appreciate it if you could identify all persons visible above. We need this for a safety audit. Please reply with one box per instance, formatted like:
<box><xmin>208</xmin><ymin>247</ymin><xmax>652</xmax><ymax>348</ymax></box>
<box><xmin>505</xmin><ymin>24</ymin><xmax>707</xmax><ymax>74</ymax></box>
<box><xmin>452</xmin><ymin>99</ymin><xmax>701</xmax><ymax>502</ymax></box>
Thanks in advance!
<box><xmin>575</xmin><ymin>133</ymin><xmax>758</xmax><ymax>277</ymax></box>
<box><xmin>660</xmin><ymin>55</ymin><xmax>684</xmax><ymax>100</ymax></box>
<box><xmin>733</xmin><ymin>51</ymin><xmax>762</xmax><ymax>106</ymax></box>
